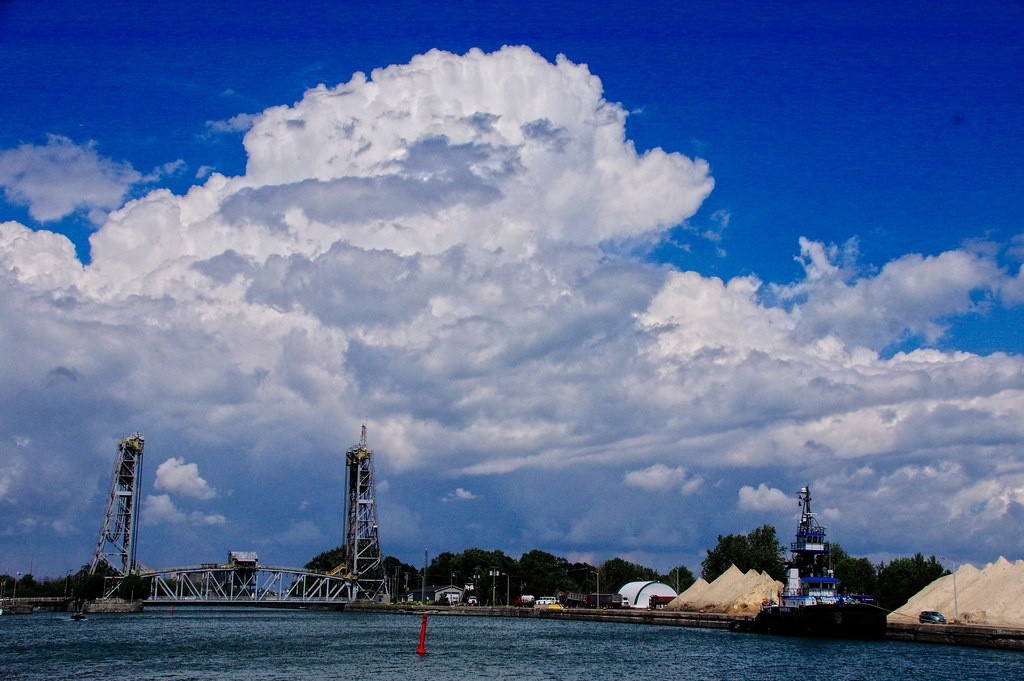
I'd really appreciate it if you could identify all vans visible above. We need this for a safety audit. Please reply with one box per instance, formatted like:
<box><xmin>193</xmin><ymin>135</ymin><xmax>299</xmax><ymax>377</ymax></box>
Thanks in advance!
<box><xmin>535</xmin><ymin>597</ymin><xmax>556</xmax><ymax>605</ymax></box>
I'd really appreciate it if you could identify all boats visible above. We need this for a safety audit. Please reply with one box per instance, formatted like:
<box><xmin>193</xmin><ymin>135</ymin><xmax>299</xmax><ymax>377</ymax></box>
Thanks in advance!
<box><xmin>729</xmin><ymin>482</ymin><xmax>888</xmax><ymax>643</ymax></box>
<box><xmin>71</xmin><ymin>613</ymin><xmax>86</xmax><ymax>619</ymax></box>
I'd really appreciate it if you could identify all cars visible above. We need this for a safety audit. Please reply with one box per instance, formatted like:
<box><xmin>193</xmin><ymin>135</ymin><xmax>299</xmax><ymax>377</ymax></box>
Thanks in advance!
<box><xmin>468</xmin><ymin>597</ymin><xmax>477</xmax><ymax>606</ymax></box>
<box><xmin>919</xmin><ymin>611</ymin><xmax>945</xmax><ymax>625</ymax></box>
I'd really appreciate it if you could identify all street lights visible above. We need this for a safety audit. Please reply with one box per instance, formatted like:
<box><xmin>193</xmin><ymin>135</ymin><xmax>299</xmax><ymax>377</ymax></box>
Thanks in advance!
<box><xmin>668</xmin><ymin>565</ymin><xmax>680</xmax><ymax>610</ymax></box>
<box><xmin>936</xmin><ymin>554</ymin><xmax>958</xmax><ymax>624</ymax></box>
<box><xmin>589</xmin><ymin>570</ymin><xmax>600</xmax><ymax>609</ymax></box>
<box><xmin>502</xmin><ymin>572</ymin><xmax>510</xmax><ymax>606</ymax></box>
